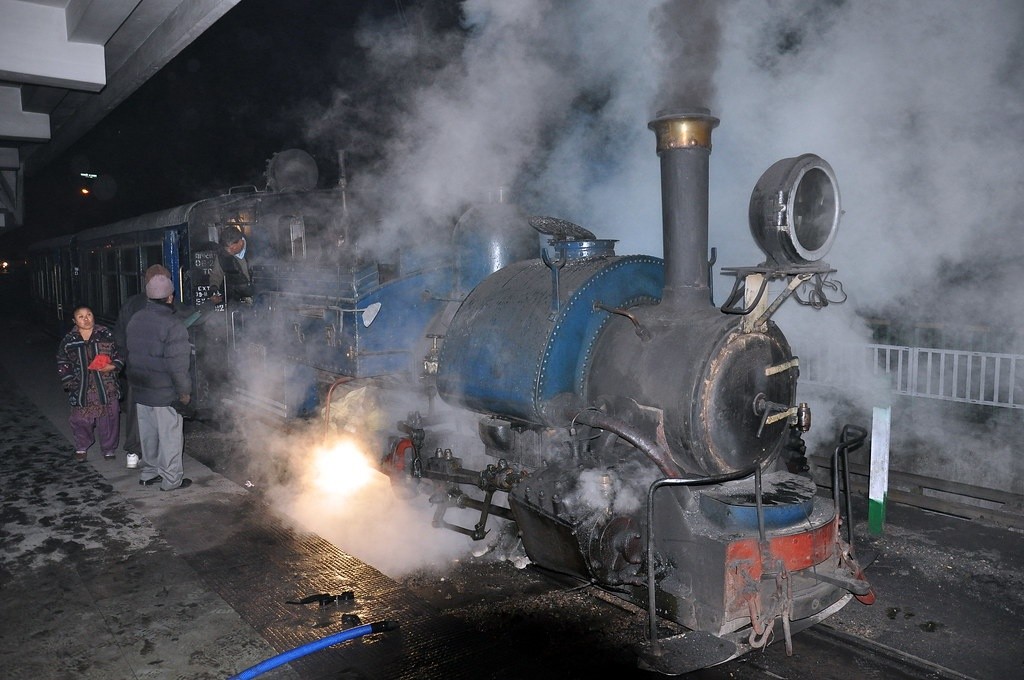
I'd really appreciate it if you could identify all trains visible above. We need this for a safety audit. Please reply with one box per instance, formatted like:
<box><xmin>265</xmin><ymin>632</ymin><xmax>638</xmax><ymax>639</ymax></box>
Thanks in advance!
<box><xmin>20</xmin><ymin>106</ymin><xmax>880</xmax><ymax>679</ymax></box>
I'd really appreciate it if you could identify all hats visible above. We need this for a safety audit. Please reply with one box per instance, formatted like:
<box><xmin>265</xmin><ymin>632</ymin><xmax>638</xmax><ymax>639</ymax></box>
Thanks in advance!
<box><xmin>145</xmin><ymin>274</ymin><xmax>174</xmax><ymax>299</ymax></box>
<box><xmin>144</xmin><ymin>264</ymin><xmax>171</xmax><ymax>286</ymax></box>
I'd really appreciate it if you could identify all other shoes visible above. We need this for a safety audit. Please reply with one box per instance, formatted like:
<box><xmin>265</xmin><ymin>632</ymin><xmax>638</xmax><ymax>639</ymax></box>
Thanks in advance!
<box><xmin>74</xmin><ymin>452</ymin><xmax>87</xmax><ymax>463</ymax></box>
<box><xmin>139</xmin><ymin>475</ymin><xmax>163</xmax><ymax>485</ymax></box>
<box><xmin>126</xmin><ymin>453</ymin><xmax>140</xmax><ymax>468</ymax></box>
<box><xmin>160</xmin><ymin>478</ymin><xmax>192</xmax><ymax>491</ymax></box>
<box><xmin>104</xmin><ymin>454</ymin><xmax>116</xmax><ymax>461</ymax></box>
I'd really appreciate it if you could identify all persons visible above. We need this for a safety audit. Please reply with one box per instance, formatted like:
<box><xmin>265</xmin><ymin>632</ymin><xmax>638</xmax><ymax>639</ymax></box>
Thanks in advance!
<box><xmin>210</xmin><ymin>227</ymin><xmax>255</xmax><ymax>331</ymax></box>
<box><xmin>57</xmin><ymin>306</ymin><xmax>125</xmax><ymax>463</ymax></box>
<box><xmin>126</xmin><ymin>275</ymin><xmax>192</xmax><ymax>491</ymax></box>
<box><xmin>114</xmin><ymin>264</ymin><xmax>222</xmax><ymax>468</ymax></box>
